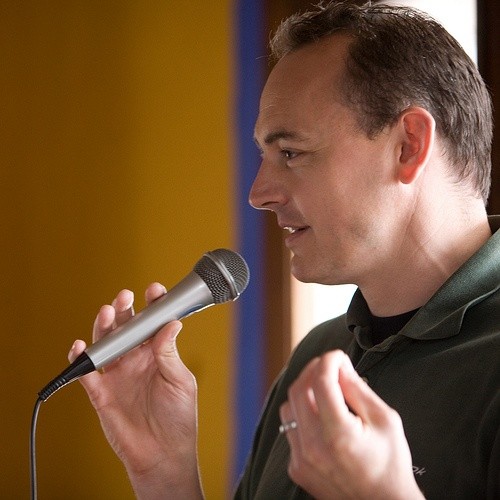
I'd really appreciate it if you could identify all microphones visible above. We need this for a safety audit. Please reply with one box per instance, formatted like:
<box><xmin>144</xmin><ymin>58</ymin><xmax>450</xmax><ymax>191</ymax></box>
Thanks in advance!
<box><xmin>39</xmin><ymin>248</ymin><xmax>250</xmax><ymax>402</ymax></box>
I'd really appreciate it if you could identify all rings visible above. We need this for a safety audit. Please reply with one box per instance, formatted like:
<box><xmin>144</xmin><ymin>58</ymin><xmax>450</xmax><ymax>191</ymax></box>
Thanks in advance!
<box><xmin>278</xmin><ymin>420</ymin><xmax>298</xmax><ymax>434</ymax></box>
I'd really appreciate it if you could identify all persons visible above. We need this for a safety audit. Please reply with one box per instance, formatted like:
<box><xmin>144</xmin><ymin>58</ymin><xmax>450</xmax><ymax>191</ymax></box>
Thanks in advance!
<box><xmin>67</xmin><ymin>0</ymin><xmax>500</xmax><ymax>500</ymax></box>
